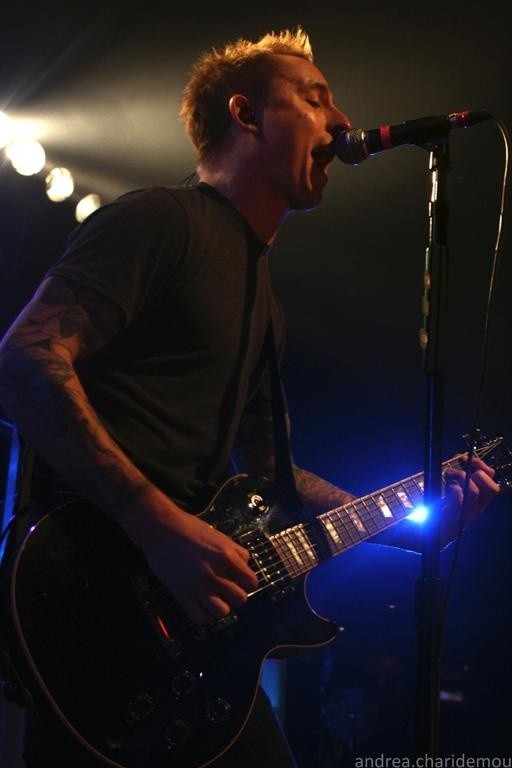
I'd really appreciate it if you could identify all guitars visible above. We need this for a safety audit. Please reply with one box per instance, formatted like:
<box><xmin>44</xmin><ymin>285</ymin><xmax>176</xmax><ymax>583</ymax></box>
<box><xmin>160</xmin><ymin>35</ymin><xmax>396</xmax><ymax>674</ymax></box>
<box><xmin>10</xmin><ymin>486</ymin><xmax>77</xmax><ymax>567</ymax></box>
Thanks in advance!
<box><xmin>1</xmin><ymin>429</ymin><xmax>512</xmax><ymax>768</ymax></box>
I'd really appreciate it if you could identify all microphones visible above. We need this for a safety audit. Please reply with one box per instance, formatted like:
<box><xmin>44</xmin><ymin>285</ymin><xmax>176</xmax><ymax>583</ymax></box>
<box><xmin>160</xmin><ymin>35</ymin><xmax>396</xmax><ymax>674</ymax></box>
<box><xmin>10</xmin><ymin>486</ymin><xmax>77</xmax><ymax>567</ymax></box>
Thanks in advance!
<box><xmin>333</xmin><ymin>109</ymin><xmax>491</xmax><ymax>165</ymax></box>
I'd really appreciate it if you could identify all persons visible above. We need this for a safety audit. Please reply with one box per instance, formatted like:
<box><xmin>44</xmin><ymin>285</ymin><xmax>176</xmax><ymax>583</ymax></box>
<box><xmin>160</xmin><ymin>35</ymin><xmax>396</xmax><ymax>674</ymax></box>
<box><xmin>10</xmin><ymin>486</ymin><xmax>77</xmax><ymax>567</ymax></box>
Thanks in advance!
<box><xmin>1</xmin><ymin>26</ymin><xmax>500</xmax><ymax>768</ymax></box>
